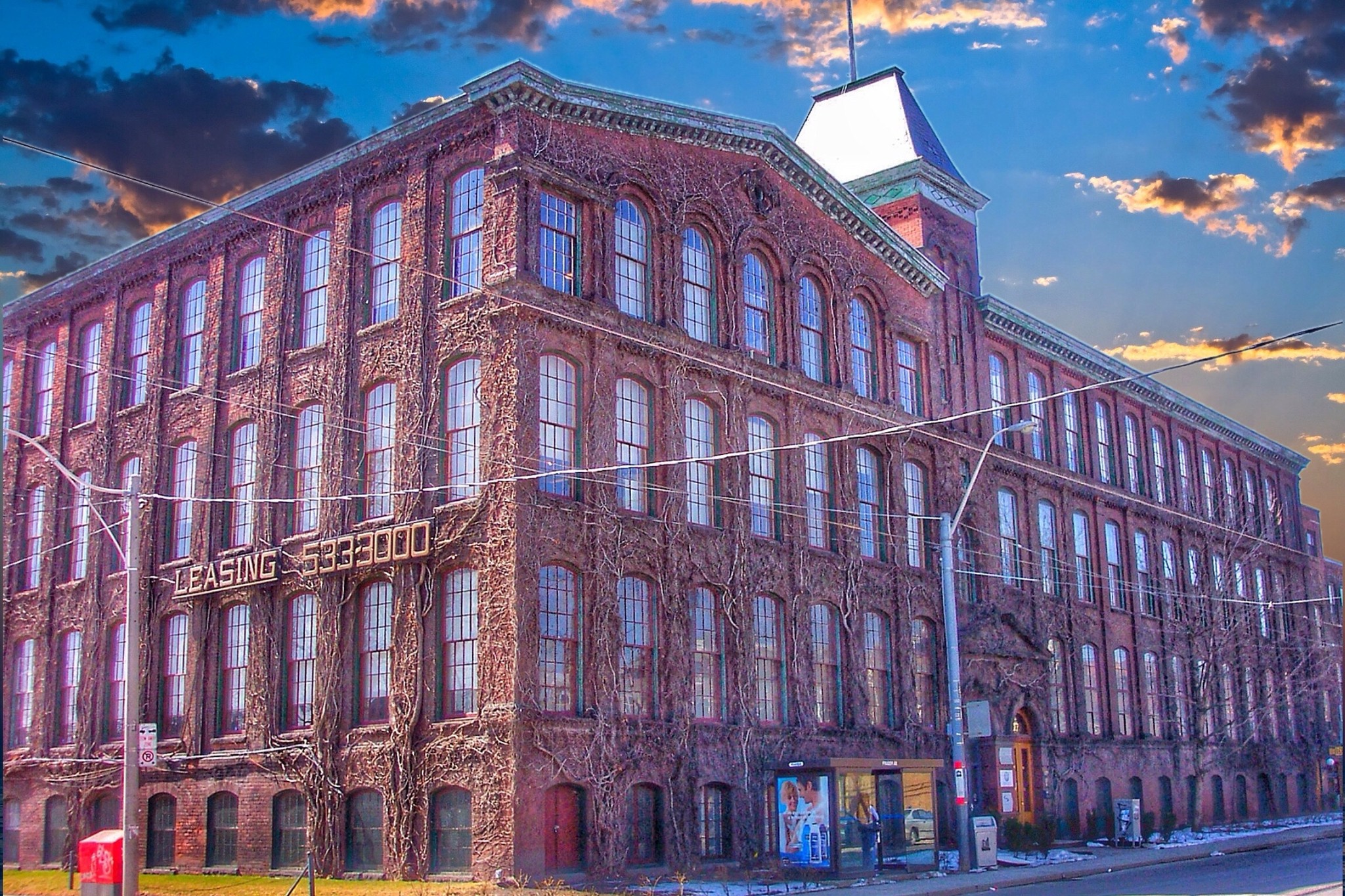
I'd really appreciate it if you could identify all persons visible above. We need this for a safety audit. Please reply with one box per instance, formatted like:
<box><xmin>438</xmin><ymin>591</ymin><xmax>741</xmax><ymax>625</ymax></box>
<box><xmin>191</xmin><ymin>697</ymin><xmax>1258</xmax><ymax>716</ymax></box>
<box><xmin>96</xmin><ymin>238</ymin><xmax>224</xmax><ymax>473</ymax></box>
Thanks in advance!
<box><xmin>779</xmin><ymin>773</ymin><xmax>823</xmax><ymax>865</ymax></box>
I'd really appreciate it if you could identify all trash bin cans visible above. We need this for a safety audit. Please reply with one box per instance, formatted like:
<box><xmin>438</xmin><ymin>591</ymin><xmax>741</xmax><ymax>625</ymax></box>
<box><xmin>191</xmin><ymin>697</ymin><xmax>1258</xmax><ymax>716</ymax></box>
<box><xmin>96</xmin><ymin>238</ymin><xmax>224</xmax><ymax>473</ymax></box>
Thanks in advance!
<box><xmin>969</xmin><ymin>816</ymin><xmax>997</xmax><ymax>868</ymax></box>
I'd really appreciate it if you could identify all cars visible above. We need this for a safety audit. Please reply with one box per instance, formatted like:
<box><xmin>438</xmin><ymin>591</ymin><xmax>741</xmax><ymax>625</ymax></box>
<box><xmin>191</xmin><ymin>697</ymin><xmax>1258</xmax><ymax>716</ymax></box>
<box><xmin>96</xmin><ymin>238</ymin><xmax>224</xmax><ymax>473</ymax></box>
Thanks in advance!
<box><xmin>904</xmin><ymin>807</ymin><xmax>935</xmax><ymax>843</ymax></box>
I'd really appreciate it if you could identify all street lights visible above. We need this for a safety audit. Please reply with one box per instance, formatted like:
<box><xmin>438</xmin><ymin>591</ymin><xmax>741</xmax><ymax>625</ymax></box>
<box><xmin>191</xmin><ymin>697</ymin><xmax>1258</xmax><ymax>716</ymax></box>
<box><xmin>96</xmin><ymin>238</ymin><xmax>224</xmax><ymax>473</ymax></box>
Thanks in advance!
<box><xmin>939</xmin><ymin>419</ymin><xmax>1039</xmax><ymax>874</ymax></box>
